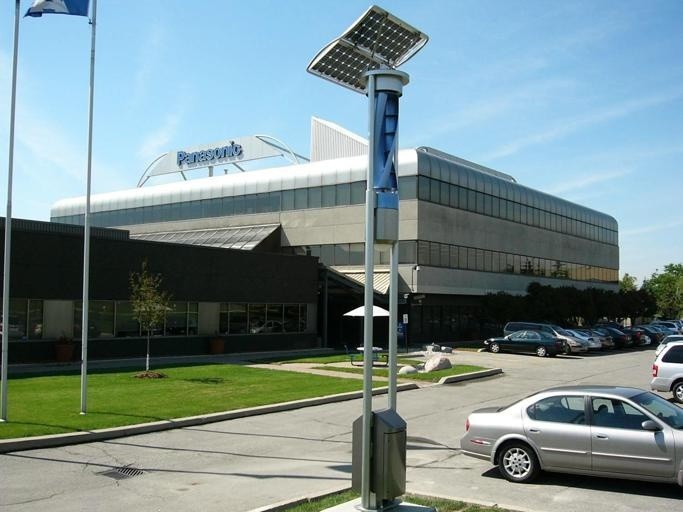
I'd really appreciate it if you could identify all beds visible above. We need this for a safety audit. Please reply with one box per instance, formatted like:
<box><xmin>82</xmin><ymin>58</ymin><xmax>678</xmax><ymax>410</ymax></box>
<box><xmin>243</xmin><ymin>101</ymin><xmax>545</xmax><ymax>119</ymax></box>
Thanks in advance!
<box><xmin>342</xmin><ymin>305</ymin><xmax>389</xmax><ymax>317</ymax></box>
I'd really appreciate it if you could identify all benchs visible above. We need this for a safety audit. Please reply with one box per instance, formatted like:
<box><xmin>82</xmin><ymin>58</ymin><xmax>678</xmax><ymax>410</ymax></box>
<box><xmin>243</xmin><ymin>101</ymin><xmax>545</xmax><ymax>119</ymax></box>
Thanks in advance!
<box><xmin>22</xmin><ymin>0</ymin><xmax>90</xmax><ymax>18</ymax></box>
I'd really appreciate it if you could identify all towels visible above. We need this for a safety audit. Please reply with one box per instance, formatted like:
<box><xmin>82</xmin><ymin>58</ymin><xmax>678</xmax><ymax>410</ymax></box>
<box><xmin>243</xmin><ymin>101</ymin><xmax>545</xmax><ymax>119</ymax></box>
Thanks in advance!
<box><xmin>352</xmin><ymin>408</ymin><xmax>406</xmax><ymax>511</ymax></box>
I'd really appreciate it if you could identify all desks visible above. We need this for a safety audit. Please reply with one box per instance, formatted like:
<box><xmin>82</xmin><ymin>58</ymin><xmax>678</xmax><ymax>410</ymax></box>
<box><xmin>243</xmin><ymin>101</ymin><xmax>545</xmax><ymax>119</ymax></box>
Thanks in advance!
<box><xmin>306</xmin><ymin>3</ymin><xmax>429</xmax><ymax>509</ymax></box>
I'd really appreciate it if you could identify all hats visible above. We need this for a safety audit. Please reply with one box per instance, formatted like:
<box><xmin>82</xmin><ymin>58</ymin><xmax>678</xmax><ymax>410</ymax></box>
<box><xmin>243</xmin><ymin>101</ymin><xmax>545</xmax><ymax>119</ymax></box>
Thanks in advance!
<box><xmin>483</xmin><ymin>322</ymin><xmax>651</xmax><ymax>357</ymax></box>
<box><xmin>650</xmin><ymin>334</ymin><xmax>683</xmax><ymax>404</ymax></box>
<box><xmin>459</xmin><ymin>385</ymin><xmax>683</xmax><ymax>486</ymax></box>
<box><xmin>631</xmin><ymin>320</ymin><xmax>683</xmax><ymax>347</ymax></box>
